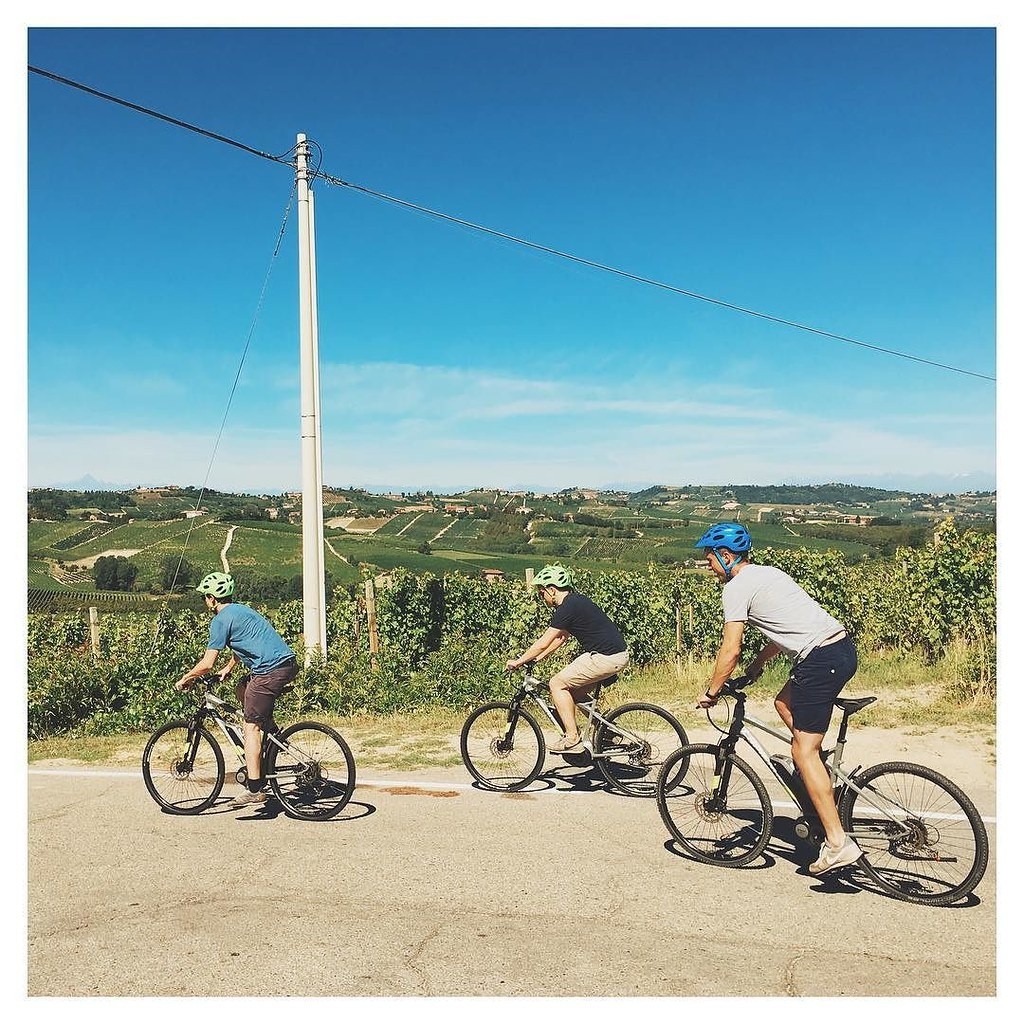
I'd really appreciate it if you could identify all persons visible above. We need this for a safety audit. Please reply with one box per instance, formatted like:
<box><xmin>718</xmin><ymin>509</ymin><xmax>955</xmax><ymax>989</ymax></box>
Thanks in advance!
<box><xmin>506</xmin><ymin>566</ymin><xmax>630</xmax><ymax>752</ymax></box>
<box><xmin>175</xmin><ymin>572</ymin><xmax>299</xmax><ymax>806</ymax></box>
<box><xmin>696</xmin><ymin>523</ymin><xmax>863</xmax><ymax>874</ymax></box>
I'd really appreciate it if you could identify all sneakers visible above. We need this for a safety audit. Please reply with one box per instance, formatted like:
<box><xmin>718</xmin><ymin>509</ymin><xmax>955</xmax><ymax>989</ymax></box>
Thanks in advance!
<box><xmin>227</xmin><ymin>786</ymin><xmax>268</xmax><ymax>807</ymax></box>
<box><xmin>809</xmin><ymin>834</ymin><xmax>864</xmax><ymax>876</ymax></box>
<box><xmin>545</xmin><ymin>733</ymin><xmax>585</xmax><ymax>753</ymax></box>
<box><xmin>604</xmin><ymin>731</ymin><xmax>624</xmax><ymax>746</ymax></box>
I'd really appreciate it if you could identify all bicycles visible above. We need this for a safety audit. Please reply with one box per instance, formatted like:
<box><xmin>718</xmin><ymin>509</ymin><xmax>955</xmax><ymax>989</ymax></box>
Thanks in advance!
<box><xmin>141</xmin><ymin>673</ymin><xmax>356</xmax><ymax>821</ymax></box>
<box><xmin>656</xmin><ymin>668</ymin><xmax>989</xmax><ymax>908</ymax></box>
<box><xmin>459</xmin><ymin>653</ymin><xmax>691</xmax><ymax>799</ymax></box>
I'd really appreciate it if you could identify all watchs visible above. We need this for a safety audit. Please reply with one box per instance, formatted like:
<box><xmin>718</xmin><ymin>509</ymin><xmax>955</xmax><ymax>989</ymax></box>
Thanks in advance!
<box><xmin>706</xmin><ymin>687</ymin><xmax>718</xmax><ymax>699</ymax></box>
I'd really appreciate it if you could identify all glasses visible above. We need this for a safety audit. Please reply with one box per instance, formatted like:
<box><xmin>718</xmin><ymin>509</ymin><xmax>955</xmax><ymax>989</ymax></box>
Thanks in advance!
<box><xmin>539</xmin><ymin>584</ymin><xmax>558</xmax><ymax>599</ymax></box>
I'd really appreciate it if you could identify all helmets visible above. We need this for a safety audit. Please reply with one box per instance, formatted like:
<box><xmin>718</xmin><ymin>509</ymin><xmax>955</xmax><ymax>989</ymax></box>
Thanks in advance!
<box><xmin>531</xmin><ymin>566</ymin><xmax>573</xmax><ymax>587</ymax></box>
<box><xmin>694</xmin><ymin>523</ymin><xmax>751</xmax><ymax>551</ymax></box>
<box><xmin>196</xmin><ymin>572</ymin><xmax>234</xmax><ymax>598</ymax></box>
